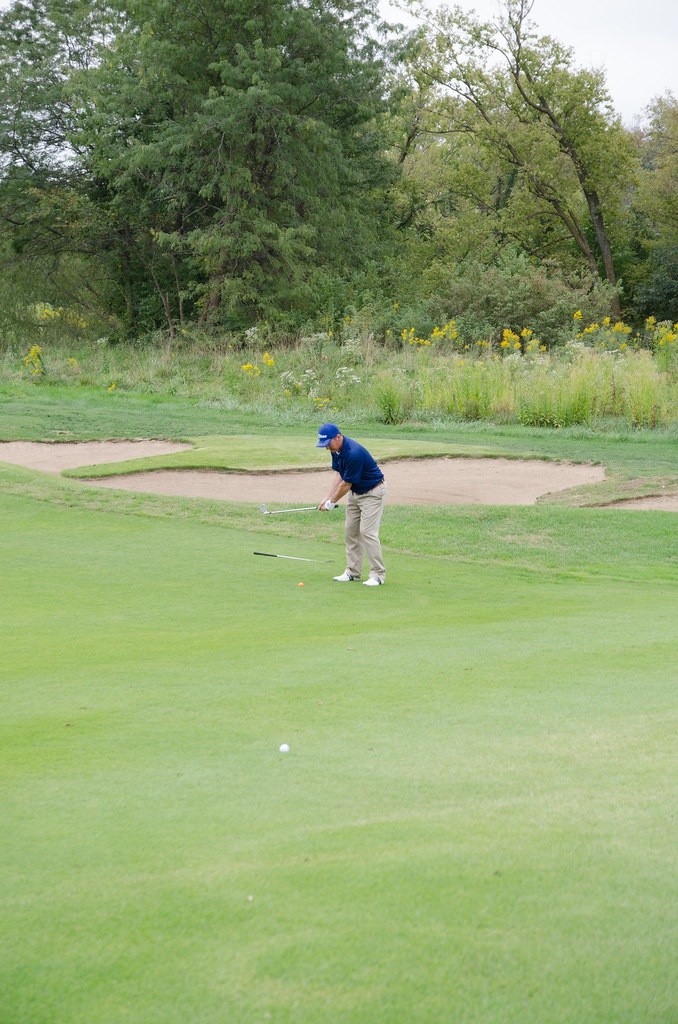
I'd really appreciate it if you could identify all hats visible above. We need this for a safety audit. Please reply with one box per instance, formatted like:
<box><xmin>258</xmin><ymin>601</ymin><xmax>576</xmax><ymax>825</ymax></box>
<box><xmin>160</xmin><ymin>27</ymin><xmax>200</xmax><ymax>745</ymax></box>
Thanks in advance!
<box><xmin>316</xmin><ymin>424</ymin><xmax>340</xmax><ymax>447</ymax></box>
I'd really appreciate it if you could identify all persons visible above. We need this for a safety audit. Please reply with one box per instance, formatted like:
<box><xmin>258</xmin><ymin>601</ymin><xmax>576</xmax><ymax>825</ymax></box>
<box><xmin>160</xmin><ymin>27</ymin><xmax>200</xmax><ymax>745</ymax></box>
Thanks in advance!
<box><xmin>316</xmin><ymin>424</ymin><xmax>386</xmax><ymax>587</ymax></box>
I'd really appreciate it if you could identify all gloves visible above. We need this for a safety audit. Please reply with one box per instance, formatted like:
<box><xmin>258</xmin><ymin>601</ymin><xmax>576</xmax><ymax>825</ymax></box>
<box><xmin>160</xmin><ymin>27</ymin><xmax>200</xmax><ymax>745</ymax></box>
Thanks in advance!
<box><xmin>326</xmin><ymin>499</ymin><xmax>336</xmax><ymax>511</ymax></box>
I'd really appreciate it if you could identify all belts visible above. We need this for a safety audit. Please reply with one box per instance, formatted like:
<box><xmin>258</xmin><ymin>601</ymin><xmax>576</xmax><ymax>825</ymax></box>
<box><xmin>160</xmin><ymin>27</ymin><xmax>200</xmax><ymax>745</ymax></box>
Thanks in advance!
<box><xmin>368</xmin><ymin>479</ymin><xmax>384</xmax><ymax>491</ymax></box>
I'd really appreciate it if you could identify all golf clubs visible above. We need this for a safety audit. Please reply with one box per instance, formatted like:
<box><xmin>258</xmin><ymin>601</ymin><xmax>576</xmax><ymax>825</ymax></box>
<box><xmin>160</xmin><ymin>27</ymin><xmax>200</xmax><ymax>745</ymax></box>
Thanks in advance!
<box><xmin>253</xmin><ymin>551</ymin><xmax>333</xmax><ymax>562</ymax></box>
<box><xmin>260</xmin><ymin>503</ymin><xmax>338</xmax><ymax>514</ymax></box>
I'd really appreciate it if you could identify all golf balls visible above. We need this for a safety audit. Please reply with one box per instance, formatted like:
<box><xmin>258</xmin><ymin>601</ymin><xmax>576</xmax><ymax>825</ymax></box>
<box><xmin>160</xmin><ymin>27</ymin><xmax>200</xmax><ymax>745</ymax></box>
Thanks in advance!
<box><xmin>299</xmin><ymin>583</ymin><xmax>303</xmax><ymax>586</ymax></box>
<box><xmin>279</xmin><ymin>744</ymin><xmax>290</xmax><ymax>753</ymax></box>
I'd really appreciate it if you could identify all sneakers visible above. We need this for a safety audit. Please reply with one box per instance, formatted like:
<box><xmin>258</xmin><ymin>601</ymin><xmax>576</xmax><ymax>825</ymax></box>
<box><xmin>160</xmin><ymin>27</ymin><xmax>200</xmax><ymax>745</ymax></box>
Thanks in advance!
<box><xmin>362</xmin><ymin>577</ymin><xmax>384</xmax><ymax>586</ymax></box>
<box><xmin>334</xmin><ymin>571</ymin><xmax>361</xmax><ymax>581</ymax></box>
